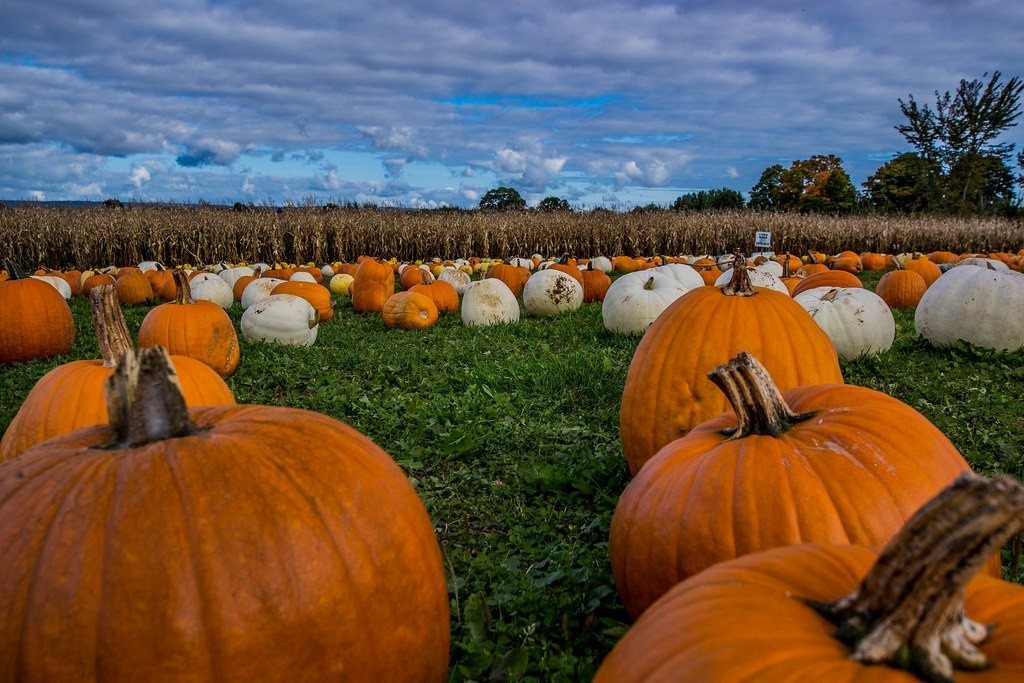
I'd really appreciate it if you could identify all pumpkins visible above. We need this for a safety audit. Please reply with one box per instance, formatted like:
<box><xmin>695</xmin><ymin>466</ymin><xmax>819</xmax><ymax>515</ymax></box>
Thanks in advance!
<box><xmin>0</xmin><ymin>250</ymin><xmax>1024</xmax><ymax>683</ymax></box>
